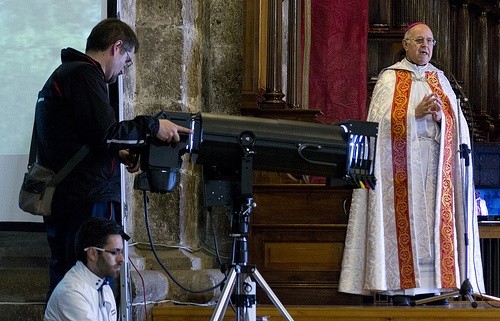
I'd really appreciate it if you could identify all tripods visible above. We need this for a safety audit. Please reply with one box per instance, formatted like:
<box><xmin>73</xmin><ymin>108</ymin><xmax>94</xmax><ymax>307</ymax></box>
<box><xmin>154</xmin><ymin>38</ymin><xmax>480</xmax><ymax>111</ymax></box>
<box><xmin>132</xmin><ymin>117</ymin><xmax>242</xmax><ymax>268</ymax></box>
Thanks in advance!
<box><xmin>410</xmin><ymin>94</ymin><xmax>500</xmax><ymax>308</ymax></box>
<box><xmin>209</xmin><ymin>199</ymin><xmax>293</xmax><ymax>321</ymax></box>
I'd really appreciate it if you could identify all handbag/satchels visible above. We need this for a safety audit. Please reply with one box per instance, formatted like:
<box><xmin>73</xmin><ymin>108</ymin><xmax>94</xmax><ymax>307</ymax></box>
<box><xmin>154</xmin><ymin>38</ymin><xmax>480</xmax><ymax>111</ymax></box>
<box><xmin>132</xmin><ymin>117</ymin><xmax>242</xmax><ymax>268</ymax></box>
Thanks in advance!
<box><xmin>19</xmin><ymin>161</ymin><xmax>55</xmax><ymax>215</ymax></box>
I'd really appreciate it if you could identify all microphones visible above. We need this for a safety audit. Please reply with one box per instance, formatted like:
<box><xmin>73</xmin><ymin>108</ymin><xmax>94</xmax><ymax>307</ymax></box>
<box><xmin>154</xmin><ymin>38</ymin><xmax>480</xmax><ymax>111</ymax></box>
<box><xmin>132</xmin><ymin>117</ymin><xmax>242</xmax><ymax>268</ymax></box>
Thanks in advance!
<box><xmin>456</xmin><ymin>85</ymin><xmax>469</xmax><ymax>102</ymax></box>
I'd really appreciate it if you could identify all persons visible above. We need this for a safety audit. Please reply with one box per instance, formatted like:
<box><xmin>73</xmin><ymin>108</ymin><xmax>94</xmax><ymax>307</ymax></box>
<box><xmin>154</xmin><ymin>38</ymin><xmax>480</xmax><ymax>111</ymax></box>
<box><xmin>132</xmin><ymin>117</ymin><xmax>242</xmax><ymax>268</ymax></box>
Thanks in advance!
<box><xmin>40</xmin><ymin>217</ymin><xmax>126</xmax><ymax>321</ymax></box>
<box><xmin>18</xmin><ymin>16</ymin><xmax>196</xmax><ymax>321</ymax></box>
<box><xmin>337</xmin><ymin>21</ymin><xmax>491</xmax><ymax>309</ymax></box>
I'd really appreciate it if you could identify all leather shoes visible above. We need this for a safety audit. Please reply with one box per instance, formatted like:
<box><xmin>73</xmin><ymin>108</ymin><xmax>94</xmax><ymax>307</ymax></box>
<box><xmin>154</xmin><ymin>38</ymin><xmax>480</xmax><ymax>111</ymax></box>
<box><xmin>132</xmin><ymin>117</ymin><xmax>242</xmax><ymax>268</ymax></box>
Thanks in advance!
<box><xmin>414</xmin><ymin>294</ymin><xmax>448</xmax><ymax>305</ymax></box>
<box><xmin>392</xmin><ymin>295</ymin><xmax>411</xmax><ymax>305</ymax></box>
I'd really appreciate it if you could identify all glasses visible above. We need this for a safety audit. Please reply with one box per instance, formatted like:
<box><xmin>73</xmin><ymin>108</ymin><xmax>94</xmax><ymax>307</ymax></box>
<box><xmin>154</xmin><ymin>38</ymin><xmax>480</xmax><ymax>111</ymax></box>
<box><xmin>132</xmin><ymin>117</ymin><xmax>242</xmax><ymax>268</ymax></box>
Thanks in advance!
<box><xmin>84</xmin><ymin>247</ymin><xmax>125</xmax><ymax>256</ymax></box>
<box><xmin>407</xmin><ymin>38</ymin><xmax>436</xmax><ymax>45</ymax></box>
<box><xmin>122</xmin><ymin>45</ymin><xmax>133</xmax><ymax>69</ymax></box>
